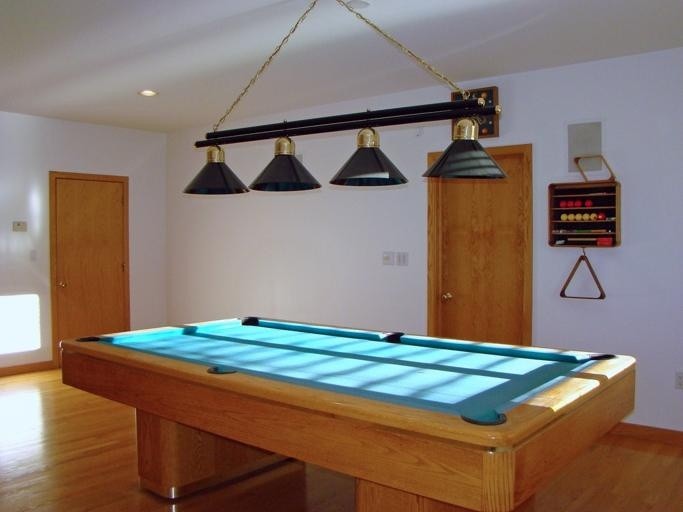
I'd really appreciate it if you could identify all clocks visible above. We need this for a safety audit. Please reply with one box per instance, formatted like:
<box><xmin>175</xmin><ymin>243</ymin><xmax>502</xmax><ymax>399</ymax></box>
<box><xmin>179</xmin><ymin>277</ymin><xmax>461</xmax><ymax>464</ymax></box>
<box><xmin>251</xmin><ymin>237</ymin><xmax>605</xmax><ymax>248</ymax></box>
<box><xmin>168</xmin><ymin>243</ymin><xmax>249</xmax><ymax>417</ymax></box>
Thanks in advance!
<box><xmin>451</xmin><ymin>86</ymin><xmax>500</xmax><ymax>140</ymax></box>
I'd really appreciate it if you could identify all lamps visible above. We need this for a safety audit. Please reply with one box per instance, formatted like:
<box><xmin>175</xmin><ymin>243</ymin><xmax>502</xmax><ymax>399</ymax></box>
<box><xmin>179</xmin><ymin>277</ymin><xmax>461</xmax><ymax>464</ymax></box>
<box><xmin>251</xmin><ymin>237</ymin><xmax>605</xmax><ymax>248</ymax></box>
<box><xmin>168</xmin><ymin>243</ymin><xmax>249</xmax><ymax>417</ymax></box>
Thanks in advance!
<box><xmin>180</xmin><ymin>0</ymin><xmax>507</xmax><ymax>195</ymax></box>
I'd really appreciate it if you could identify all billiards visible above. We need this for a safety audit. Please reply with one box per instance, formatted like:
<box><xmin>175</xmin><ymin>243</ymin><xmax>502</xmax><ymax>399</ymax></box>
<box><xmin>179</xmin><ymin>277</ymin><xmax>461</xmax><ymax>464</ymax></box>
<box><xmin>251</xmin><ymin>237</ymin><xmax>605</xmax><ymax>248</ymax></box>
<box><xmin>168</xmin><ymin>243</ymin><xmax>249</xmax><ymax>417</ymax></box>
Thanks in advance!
<box><xmin>559</xmin><ymin>200</ymin><xmax>592</xmax><ymax>207</ymax></box>
<box><xmin>561</xmin><ymin>212</ymin><xmax>606</xmax><ymax>220</ymax></box>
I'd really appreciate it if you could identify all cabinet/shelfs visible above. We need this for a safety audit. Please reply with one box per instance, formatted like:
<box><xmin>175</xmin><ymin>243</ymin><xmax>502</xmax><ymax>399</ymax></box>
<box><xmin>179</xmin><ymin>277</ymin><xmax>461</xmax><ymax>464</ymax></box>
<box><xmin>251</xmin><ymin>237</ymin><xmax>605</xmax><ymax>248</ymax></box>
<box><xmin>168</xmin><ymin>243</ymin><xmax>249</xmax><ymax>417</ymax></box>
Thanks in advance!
<box><xmin>547</xmin><ymin>179</ymin><xmax>622</xmax><ymax>248</ymax></box>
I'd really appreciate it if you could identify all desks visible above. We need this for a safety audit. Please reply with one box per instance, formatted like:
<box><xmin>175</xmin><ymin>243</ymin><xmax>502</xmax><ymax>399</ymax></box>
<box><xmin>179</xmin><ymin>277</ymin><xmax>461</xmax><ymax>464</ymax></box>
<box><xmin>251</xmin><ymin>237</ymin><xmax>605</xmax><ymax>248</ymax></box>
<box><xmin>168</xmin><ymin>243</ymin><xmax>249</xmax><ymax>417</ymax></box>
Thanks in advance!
<box><xmin>59</xmin><ymin>314</ymin><xmax>637</xmax><ymax>512</ymax></box>
